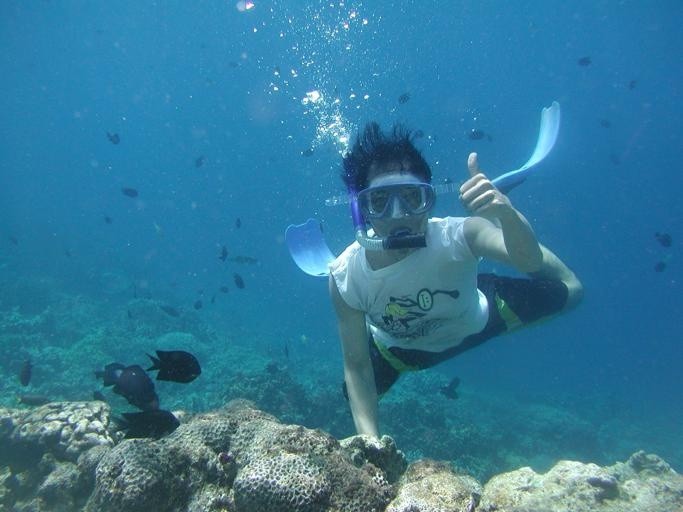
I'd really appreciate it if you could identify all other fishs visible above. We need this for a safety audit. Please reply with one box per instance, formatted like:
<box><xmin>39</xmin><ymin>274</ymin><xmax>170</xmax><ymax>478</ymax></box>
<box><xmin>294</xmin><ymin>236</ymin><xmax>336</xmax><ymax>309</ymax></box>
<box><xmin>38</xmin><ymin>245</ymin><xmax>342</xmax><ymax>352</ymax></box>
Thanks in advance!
<box><xmin>189</xmin><ymin>275</ymin><xmax>245</xmax><ymax>311</ymax></box>
<box><xmin>158</xmin><ymin>301</ymin><xmax>180</xmax><ymax>318</ymax></box>
<box><xmin>17</xmin><ymin>396</ymin><xmax>49</xmax><ymax>406</ymax></box>
<box><xmin>147</xmin><ymin>349</ymin><xmax>202</xmax><ymax>385</ymax></box>
<box><xmin>22</xmin><ymin>356</ymin><xmax>32</xmax><ymax>386</ymax></box>
<box><xmin>440</xmin><ymin>377</ymin><xmax>462</xmax><ymax>399</ymax></box>
<box><xmin>123</xmin><ymin>187</ymin><xmax>141</xmax><ymax>199</ymax></box>
<box><xmin>89</xmin><ymin>390</ymin><xmax>106</xmax><ymax>401</ymax></box>
<box><xmin>112</xmin><ymin>407</ymin><xmax>180</xmax><ymax>440</ymax></box>
<box><xmin>107</xmin><ymin>131</ymin><xmax>122</xmax><ymax>145</ymax></box>
<box><xmin>656</xmin><ymin>232</ymin><xmax>675</xmax><ymax>247</ymax></box>
<box><xmin>112</xmin><ymin>364</ymin><xmax>160</xmax><ymax>410</ymax></box>
<box><xmin>220</xmin><ymin>244</ymin><xmax>228</xmax><ymax>263</ymax></box>
<box><xmin>653</xmin><ymin>260</ymin><xmax>666</xmax><ymax>272</ymax></box>
<box><xmin>94</xmin><ymin>362</ymin><xmax>123</xmax><ymax>387</ymax></box>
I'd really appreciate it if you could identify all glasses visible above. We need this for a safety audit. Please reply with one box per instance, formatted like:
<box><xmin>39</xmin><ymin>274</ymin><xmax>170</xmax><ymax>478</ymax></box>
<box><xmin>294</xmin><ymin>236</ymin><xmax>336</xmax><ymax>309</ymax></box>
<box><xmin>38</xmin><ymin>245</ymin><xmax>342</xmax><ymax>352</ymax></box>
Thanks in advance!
<box><xmin>350</xmin><ymin>180</ymin><xmax>437</xmax><ymax>220</ymax></box>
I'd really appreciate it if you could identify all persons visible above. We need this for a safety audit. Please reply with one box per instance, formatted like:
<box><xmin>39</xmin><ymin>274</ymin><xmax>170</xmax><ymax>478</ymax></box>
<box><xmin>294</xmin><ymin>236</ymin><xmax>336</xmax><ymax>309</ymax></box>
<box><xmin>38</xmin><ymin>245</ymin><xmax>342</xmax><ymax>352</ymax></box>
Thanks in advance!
<box><xmin>326</xmin><ymin>120</ymin><xmax>585</xmax><ymax>445</ymax></box>
